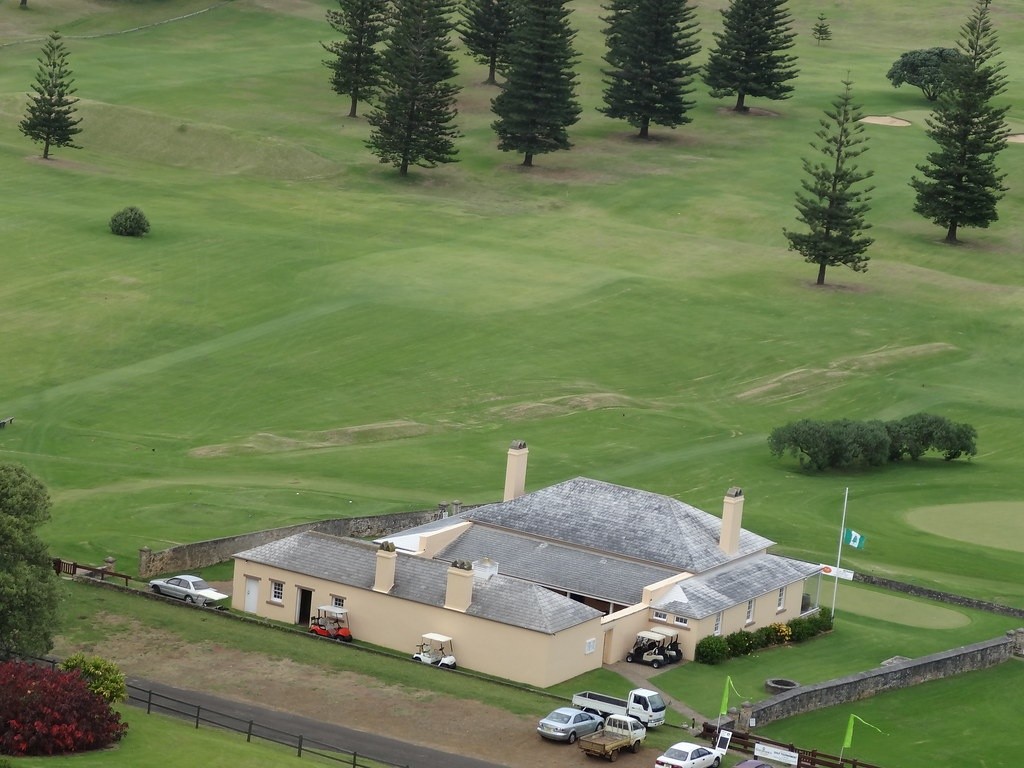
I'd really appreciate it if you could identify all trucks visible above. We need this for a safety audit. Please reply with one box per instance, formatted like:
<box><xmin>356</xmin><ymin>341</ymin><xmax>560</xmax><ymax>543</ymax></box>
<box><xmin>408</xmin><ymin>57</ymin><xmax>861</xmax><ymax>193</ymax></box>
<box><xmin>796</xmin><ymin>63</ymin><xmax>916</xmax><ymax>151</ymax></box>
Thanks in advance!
<box><xmin>572</xmin><ymin>687</ymin><xmax>667</xmax><ymax>728</ymax></box>
<box><xmin>577</xmin><ymin>714</ymin><xmax>647</xmax><ymax>762</ymax></box>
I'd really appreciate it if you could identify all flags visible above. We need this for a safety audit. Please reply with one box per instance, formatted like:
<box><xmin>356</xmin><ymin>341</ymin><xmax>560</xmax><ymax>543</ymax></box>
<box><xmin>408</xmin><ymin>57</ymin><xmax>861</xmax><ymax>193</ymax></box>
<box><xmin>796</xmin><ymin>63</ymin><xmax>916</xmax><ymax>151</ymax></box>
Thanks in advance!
<box><xmin>843</xmin><ymin>528</ymin><xmax>866</xmax><ymax>549</ymax></box>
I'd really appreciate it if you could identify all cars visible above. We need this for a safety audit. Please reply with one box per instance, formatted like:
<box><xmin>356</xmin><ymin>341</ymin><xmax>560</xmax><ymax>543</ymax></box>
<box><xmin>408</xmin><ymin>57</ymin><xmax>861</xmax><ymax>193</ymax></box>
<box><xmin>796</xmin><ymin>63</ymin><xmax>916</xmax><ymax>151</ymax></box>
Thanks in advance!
<box><xmin>536</xmin><ymin>707</ymin><xmax>604</xmax><ymax>743</ymax></box>
<box><xmin>732</xmin><ymin>759</ymin><xmax>775</xmax><ymax>768</ymax></box>
<box><xmin>148</xmin><ymin>572</ymin><xmax>229</xmax><ymax>604</ymax></box>
<box><xmin>654</xmin><ymin>741</ymin><xmax>723</xmax><ymax>768</ymax></box>
